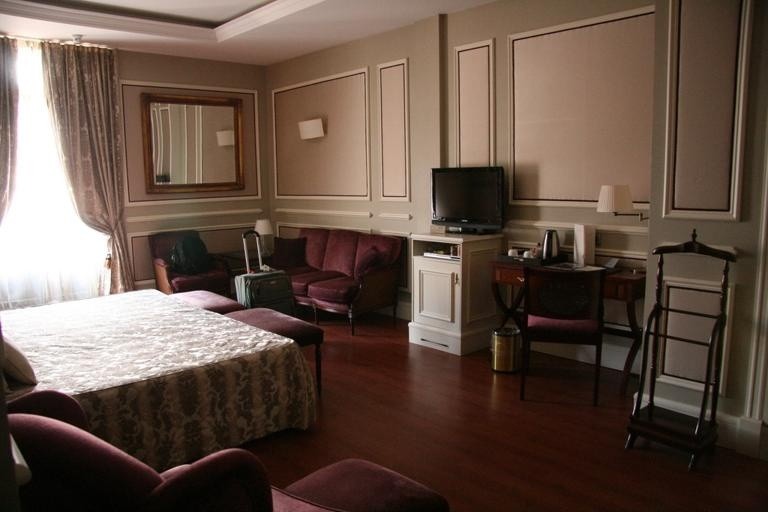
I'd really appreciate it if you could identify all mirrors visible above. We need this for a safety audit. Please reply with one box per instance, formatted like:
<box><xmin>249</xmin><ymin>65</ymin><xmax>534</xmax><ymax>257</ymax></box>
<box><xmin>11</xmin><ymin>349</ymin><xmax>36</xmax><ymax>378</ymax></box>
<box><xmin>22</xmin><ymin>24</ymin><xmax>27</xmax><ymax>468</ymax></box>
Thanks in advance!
<box><xmin>138</xmin><ymin>90</ymin><xmax>248</xmax><ymax>197</ymax></box>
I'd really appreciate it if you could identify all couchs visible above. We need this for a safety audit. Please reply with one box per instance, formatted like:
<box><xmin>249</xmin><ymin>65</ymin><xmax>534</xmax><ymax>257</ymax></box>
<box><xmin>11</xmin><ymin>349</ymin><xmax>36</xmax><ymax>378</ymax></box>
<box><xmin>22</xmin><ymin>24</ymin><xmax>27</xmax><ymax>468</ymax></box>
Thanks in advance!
<box><xmin>273</xmin><ymin>224</ymin><xmax>401</xmax><ymax>334</ymax></box>
<box><xmin>135</xmin><ymin>222</ymin><xmax>230</xmax><ymax>298</ymax></box>
<box><xmin>0</xmin><ymin>388</ymin><xmax>348</xmax><ymax>512</ymax></box>
<box><xmin>283</xmin><ymin>455</ymin><xmax>453</xmax><ymax>511</ymax></box>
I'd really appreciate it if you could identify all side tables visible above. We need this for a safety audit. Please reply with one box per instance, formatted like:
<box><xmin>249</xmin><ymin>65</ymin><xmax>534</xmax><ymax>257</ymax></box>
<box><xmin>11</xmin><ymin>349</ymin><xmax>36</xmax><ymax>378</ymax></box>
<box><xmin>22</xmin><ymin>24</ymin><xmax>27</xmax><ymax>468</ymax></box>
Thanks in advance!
<box><xmin>225</xmin><ymin>248</ymin><xmax>279</xmax><ymax>269</ymax></box>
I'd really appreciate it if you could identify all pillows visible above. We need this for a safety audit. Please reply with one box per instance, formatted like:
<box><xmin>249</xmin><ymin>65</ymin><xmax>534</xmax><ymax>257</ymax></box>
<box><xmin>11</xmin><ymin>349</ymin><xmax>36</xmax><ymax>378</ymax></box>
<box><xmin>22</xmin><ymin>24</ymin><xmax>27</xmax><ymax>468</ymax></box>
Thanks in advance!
<box><xmin>272</xmin><ymin>233</ymin><xmax>309</xmax><ymax>269</ymax></box>
<box><xmin>1</xmin><ymin>338</ymin><xmax>38</xmax><ymax>390</ymax></box>
<box><xmin>352</xmin><ymin>244</ymin><xmax>383</xmax><ymax>284</ymax></box>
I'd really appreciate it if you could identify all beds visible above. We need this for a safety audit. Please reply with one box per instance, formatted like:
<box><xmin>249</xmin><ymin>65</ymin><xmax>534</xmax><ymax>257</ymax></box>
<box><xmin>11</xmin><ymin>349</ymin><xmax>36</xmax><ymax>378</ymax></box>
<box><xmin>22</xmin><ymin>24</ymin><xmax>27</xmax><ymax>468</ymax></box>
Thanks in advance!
<box><xmin>0</xmin><ymin>289</ymin><xmax>319</xmax><ymax>469</ymax></box>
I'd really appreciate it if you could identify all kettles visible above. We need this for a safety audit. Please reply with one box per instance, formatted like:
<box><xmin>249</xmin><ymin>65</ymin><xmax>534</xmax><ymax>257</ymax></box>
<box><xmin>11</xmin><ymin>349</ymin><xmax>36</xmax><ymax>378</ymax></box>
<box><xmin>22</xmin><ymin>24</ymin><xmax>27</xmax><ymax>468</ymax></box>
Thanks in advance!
<box><xmin>540</xmin><ymin>229</ymin><xmax>561</xmax><ymax>264</ymax></box>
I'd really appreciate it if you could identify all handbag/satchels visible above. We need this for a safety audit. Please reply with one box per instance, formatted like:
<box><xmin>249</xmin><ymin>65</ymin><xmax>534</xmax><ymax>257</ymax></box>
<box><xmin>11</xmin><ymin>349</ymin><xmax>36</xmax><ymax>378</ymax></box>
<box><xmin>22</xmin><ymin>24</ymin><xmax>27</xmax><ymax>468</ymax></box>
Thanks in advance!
<box><xmin>167</xmin><ymin>237</ymin><xmax>208</xmax><ymax>275</ymax></box>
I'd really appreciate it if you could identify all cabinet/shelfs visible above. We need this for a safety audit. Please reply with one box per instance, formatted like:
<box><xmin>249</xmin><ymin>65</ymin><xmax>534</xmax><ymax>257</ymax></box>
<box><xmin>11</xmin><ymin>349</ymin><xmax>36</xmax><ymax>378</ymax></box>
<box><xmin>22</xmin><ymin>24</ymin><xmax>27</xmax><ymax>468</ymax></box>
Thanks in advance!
<box><xmin>404</xmin><ymin>230</ymin><xmax>505</xmax><ymax>357</ymax></box>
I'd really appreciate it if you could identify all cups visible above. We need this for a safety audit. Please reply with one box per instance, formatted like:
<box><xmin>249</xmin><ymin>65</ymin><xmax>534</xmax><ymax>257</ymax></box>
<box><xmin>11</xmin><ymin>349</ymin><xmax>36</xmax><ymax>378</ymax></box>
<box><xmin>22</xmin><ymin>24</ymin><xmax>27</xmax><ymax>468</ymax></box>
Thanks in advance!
<box><xmin>507</xmin><ymin>248</ymin><xmax>518</xmax><ymax>257</ymax></box>
<box><xmin>524</xmin><ymin>251</ymin><xmax>533</xmax><ymax>258</ymax></box>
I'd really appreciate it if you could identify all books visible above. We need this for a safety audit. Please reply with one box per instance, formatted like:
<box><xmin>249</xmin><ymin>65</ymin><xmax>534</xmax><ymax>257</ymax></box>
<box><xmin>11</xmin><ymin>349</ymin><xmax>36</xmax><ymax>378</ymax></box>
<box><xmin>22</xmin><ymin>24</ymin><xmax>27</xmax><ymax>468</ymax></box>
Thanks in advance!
<box><xmin>424</xmin><ymin>252</ymin><xmax>451</xmax><ymax>260</ymax></box>
<box><xmin>574</xmin><ymin>224</ymin><xmax>595</xmax><ymax>265</ymax></box>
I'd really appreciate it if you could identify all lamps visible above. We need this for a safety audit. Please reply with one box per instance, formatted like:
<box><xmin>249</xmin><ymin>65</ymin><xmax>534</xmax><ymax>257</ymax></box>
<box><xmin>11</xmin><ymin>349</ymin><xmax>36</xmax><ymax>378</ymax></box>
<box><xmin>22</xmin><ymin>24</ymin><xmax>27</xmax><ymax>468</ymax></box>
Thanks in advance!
<box><xmin>251</xmin><ymin>218</ymin><xmax>277</xmax><ymax>257</ymax></box>
<box><xmin>215</xmin><ymin>130</ymin><xmax>234</xmax><ymax>146</ymax></box>
<box><xmin>590</xmin><ymin>182</ymin><xmax>651</xmax><ymax>225</ymax></box>
<box><xmin>297</xmin><ymin>117</ymin><xmax>324</xmax><ymax>140</ymax></box>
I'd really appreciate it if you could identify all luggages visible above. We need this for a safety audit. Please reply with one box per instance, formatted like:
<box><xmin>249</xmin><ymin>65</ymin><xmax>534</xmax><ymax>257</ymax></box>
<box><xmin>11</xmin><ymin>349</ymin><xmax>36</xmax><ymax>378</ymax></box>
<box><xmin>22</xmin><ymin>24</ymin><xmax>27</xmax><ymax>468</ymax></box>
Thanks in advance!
<box><xmin>233</xmin><ymin>230</ymin><xmax>299</xmax><ymax>320</ymax></box>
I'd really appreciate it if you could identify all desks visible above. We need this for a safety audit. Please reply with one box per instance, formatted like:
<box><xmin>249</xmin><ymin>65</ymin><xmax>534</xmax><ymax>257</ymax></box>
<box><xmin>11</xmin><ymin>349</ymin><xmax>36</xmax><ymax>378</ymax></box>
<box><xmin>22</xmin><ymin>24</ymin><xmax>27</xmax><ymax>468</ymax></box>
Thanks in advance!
<box><xmin>486</xmin><ymin>255</ymin><xmax>646</xmax><ymax>401</ymax></box>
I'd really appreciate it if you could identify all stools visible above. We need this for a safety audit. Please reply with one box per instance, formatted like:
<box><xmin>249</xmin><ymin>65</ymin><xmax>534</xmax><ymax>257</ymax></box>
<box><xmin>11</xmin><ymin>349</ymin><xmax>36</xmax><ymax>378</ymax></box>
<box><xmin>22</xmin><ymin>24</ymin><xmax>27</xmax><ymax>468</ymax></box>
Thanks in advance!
<box><xmin>168</xmin><ymin>289</ymin><xmax>246</xmax><ymax>315</ymax></box>
<box><xmin>221</xmin><ymin>307</ymin><xmax>325</xmax><ymax>396</ymax></box>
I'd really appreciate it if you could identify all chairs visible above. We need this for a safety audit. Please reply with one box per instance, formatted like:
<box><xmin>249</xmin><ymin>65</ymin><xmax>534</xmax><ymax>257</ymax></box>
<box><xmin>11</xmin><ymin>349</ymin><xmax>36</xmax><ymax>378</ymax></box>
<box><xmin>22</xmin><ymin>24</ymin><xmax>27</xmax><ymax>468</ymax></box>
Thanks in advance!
<box><xmin>513</xmin><ymin>261</ymin><xmax>608</xmax><ymax>411</ymax></box>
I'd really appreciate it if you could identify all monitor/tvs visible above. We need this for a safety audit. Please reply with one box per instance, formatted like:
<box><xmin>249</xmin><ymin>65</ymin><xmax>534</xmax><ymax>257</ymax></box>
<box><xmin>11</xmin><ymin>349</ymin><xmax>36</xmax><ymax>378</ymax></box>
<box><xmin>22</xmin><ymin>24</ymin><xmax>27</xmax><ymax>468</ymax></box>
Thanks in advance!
<box><xmin>430</xmin><ymin>166</ymin><xmax>505</xmax><ymax>235</ymax></box>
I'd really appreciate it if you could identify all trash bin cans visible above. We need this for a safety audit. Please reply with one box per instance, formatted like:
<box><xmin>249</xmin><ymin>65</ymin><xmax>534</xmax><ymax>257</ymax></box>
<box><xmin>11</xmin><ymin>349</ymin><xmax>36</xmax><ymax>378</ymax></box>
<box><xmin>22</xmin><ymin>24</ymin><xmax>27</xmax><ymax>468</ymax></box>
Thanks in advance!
<box><xmin>489</xmin><ymin>326</ymin><xmax>522</xmax><ymax>375</ymax></box>
